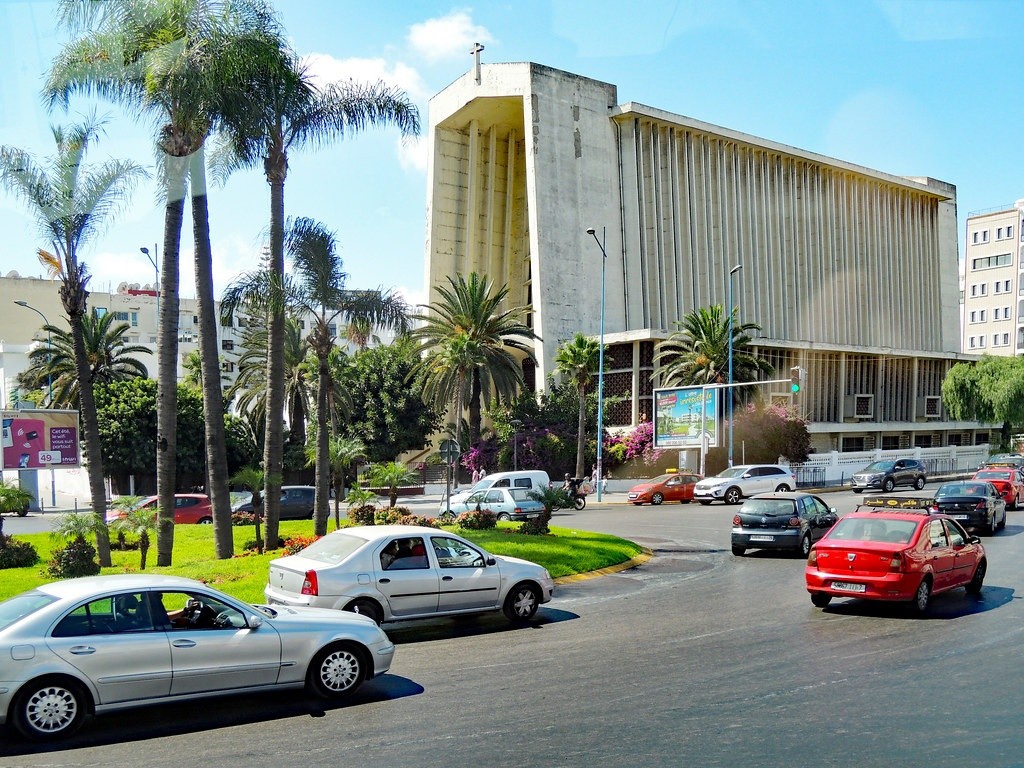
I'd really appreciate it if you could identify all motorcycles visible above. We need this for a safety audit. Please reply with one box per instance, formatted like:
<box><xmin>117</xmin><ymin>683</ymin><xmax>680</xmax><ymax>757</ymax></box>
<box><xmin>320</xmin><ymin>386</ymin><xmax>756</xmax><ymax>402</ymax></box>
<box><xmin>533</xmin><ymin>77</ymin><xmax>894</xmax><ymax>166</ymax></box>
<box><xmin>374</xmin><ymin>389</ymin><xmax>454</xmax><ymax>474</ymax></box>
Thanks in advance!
<box><xmin>550</xmin><ymin>489</ymin><xmax>587</xmax><ymax>511</ymax></box>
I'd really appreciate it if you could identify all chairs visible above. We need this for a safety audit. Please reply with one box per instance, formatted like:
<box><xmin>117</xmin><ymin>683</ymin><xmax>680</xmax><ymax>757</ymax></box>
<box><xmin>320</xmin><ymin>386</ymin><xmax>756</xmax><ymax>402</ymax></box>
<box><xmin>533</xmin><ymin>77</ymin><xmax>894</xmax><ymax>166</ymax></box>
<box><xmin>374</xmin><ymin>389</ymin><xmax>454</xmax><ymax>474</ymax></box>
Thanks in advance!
<box><xmin>115</xmin><ymin>594</ymin><xmax>150</xmax><ymax>629</ymax></box>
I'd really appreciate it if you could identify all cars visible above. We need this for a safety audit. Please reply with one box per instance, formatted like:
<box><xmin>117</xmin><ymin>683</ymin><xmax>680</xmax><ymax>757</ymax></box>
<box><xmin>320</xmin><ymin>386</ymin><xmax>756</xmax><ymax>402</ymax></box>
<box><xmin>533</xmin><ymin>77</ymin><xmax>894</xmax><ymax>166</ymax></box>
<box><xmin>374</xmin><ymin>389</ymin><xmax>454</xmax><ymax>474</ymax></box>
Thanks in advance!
<box><xmin>627</xmin><ymin>468</ymin><xmax>705</xmax><ymax>505</ymax></box>
<box><xmin>928</xmin><ymin>481</ymin><xmax>1008</xmax><ymax>534</ymax></box>
<box><xmin>977</xmin><ymin>453</ymin><xmax>1024</xmax><ymax>472</ymax></box>
<box><xmin>805</xmin><ymin>495</ymin><xmax>987</xmax><ymax>613</ymax></box>
<box><xmin>0</xmin><ymin>574</ymin><xmax>395</xmax><ymax>741</ymax></box>
<box><xmin>106</xmin><ymin>493</ymin><xmax>214</xmax><ymax>525</ymax></box>
<box><xmin>264</xmin><ymin>524</ymin><xmax>556</xmax><ymax>630</ymax></box>
<box><xmin>450</xmin><ymin>487</ymin><xmax>472</xmax><ymax>496</ymax></box>
<box><xmin>439</xmin><ymin>486</ymin><xmax>546</xmax><ymax>520</ymax></box>
<box><xmin>231</xmin><ymin>485</ymin><xmax>330</xmax><ymax>524</ymax></box>
<box><xmin>966</xmin><ymin>467</ymin><xmax>1024</xmax><ymax>509</ymax></box>
<box><xmin>731</xmin><ymin>491</ymin><xmax>840</xmax><ymax>558</ymax></box>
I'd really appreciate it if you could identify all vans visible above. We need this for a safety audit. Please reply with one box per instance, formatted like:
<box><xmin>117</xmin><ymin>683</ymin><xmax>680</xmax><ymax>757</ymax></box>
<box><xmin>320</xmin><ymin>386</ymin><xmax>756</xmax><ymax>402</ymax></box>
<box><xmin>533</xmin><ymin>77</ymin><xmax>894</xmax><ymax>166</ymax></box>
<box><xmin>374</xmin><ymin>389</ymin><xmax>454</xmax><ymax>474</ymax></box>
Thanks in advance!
<box><xmin>449</xmin><ymin>470</ymin><xmax>553</xmax><ymax>505</ymax></box>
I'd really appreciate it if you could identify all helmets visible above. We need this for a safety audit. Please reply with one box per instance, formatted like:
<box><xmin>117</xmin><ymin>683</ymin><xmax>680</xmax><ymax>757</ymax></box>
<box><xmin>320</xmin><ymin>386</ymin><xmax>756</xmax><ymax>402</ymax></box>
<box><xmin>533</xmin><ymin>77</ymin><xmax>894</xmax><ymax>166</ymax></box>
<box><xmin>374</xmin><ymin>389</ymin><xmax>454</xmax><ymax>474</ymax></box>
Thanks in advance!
<box><xmin>565</xmin><ymin>473</ymin><xmax>571</xmax><ymax>480</ymax></box>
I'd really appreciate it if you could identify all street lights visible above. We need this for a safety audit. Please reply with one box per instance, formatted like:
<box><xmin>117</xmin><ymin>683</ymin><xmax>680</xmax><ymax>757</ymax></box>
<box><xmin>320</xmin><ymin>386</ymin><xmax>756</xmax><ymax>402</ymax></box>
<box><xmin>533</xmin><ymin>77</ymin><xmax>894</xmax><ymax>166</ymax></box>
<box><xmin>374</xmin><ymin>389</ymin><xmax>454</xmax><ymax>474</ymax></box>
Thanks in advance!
<box><xmin>140</xmin><ymin>246</ymin><xmax>160</xmax><ymax>313</ymax></box>
<box><xmin>586</xmin><ymin>226</ymin><xmax>607</xmax><ymax>501</ymax></box>
<box><xmin>729</xmin><ymin>264</ymin><xmax>743</xmax><ymax>467</ymax></box>
<box><xmin>510</xmin><ymin>419</ymin><xmax>522</xmax><ymax>471</ymax></box>
<box><xmin>14</xmin><ymin>300</ymin><xmax>53</xmax><ymax>410</ymax></box>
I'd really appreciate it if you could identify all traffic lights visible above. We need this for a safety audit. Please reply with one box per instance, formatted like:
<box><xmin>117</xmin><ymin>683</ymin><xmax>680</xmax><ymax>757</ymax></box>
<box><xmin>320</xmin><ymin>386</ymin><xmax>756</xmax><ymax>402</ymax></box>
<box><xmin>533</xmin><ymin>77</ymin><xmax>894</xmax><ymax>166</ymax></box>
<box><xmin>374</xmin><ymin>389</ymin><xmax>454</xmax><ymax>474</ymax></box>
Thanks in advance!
<box><xmin>790</xmin><ymin>367</ymin><xmax>802</xmax><ymax>394</ymax></box>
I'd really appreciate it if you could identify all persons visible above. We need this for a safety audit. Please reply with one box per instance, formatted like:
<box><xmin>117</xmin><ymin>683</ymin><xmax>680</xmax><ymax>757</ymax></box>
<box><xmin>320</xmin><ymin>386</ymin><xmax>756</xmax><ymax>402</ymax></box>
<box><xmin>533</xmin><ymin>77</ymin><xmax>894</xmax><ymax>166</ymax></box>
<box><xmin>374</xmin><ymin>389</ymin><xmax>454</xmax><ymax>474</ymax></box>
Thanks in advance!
<box><xmin>279</xmin><ymin>490</ymin><xmax>288</xmax><ymax>501</ymax></box>
<box><xmin>500</xmin><ymin>481</ymin><xmax>508</xmax><ymax>486</ymax></box>
<box><xmin>563</xmin><ymin>473</ymin><xmax>575</xmax><ymax>509</ymax></box>
<box><xmin>136</xmin><ymin>593</ymin><xmax>192</xmax><ymax>627</ymax></box>
<box><xmin>590</xmin><ymin>463</ymin><xmax>608</xmax><ymax>494</ymax></box>
<box><xmin>380</xmin><ymin>539</ymin><xmax>412</xmax><ymax>571</ymax></box>
<box><xmin>472</xmin><ymin>466</ymin><xmax>486</xmax><ymax>483</ymax></box>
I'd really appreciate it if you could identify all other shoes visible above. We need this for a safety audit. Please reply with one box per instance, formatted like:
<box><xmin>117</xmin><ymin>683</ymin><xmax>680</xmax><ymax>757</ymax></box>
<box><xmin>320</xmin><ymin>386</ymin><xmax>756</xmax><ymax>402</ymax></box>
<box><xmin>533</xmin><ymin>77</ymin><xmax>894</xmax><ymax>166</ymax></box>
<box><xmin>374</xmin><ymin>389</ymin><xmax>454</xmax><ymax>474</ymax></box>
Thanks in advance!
<box><xmin>605</xmin><ymin>491</ymin><xmax>608</xmax><ymax>494</ymax></box>
<box><xmin>570</xmin><ymin>505</ymin><xmax>575</xmax><ymax>509</ymax></box>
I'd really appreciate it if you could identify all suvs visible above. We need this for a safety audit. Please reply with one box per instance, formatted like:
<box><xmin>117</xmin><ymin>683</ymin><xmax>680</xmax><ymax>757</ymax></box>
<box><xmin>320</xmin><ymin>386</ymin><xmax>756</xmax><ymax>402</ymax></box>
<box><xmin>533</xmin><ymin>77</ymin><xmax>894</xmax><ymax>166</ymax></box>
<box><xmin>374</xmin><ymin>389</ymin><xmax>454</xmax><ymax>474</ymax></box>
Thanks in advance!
<box><xmin>693</xmin><ymin>465</ymin><xmax>796</xmax><ymax>505</ymax></box>
<box><xmin>851</xmin><ymin>458</ymin><xmax>927</xmax><ymax>494</ymax></box>
